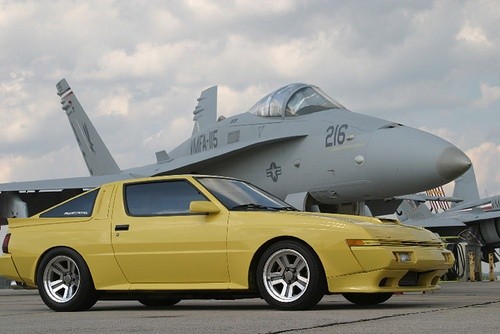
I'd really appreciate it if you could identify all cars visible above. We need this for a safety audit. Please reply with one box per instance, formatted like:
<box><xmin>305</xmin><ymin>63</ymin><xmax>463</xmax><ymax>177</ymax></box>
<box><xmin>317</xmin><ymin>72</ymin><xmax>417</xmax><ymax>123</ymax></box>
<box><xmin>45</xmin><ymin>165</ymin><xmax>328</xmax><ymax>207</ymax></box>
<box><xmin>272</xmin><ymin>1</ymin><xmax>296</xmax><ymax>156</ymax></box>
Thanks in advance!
<box><xmin>0</xmin><ymin>172</ymin><xmax>455</xmax><ymax>310</ymax></box>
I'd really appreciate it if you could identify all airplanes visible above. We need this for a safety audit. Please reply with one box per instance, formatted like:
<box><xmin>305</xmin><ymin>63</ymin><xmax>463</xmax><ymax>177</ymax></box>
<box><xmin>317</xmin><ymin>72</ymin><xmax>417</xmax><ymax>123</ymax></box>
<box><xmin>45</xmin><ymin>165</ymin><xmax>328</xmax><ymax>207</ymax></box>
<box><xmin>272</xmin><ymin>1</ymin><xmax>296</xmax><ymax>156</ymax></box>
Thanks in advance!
<box><xmin>364</xmin><ymin>162</ymin><xmax>500</xmax><ymax>263</ymax></box>
<box><xmin>1</xmin><ymin>76</ymin><xmax>500</xmax><ymax>277</ymax></box>
